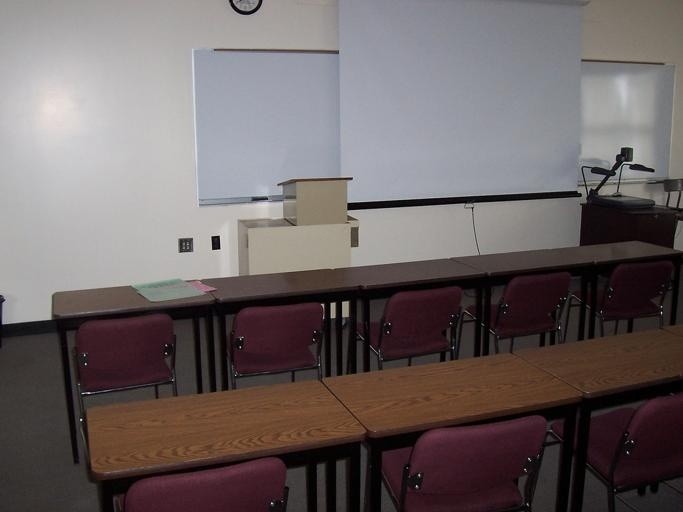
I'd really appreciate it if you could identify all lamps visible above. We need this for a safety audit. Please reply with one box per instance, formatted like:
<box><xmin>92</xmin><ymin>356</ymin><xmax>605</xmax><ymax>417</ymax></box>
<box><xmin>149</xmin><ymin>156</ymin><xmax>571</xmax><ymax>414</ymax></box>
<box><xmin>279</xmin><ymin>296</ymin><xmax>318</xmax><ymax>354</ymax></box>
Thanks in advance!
<box><xmin>589</xmin><ymin>146</ymin><xmax>634</xmax><ymax>199</ymax></box>
<box><xmin>612</xmin><ymin>163</ymin><xmax>656</xmax><ymax>197</ymax></box>
<box><xmin>580</xmin><ymin>164</ymin><xmax>617</xmax><ymax>201</ymax></box>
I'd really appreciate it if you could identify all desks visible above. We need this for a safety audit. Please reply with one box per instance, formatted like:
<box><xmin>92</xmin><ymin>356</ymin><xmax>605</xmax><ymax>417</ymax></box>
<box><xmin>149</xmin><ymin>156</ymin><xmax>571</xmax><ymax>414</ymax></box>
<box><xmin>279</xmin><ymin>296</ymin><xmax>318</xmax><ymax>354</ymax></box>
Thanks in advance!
<box><xmin>580</xmin><ymin>195</ymin><xmax>683</xmax><ymax>250</ymax></box>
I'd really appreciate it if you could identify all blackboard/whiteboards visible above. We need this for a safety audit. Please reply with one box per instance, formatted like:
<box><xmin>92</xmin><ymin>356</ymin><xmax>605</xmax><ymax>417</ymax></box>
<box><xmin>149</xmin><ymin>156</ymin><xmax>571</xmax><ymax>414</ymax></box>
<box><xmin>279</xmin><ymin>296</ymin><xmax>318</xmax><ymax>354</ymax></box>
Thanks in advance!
<box><xmin>193</xmin><ymin>49</ymin><xmax>675</xmax><ymax>206</ymax></box>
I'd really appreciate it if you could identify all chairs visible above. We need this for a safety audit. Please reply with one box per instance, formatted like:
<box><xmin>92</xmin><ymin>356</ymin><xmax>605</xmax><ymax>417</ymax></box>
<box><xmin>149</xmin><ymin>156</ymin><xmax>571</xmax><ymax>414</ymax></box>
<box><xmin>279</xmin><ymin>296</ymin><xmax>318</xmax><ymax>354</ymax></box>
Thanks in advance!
<box><xmin>662</xmin><ymin>178</ymin><xmax>683</xmax><ymax>209</ymax></box>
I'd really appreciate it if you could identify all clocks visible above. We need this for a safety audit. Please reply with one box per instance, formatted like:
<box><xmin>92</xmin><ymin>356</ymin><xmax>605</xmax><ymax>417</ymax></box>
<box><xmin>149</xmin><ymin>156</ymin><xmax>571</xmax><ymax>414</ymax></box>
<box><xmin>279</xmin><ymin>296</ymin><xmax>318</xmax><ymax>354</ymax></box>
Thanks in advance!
<box><xmin>228</xmin><ymin>0</ymin><xmax>263</xmax><ymax>16</ymax></box>
